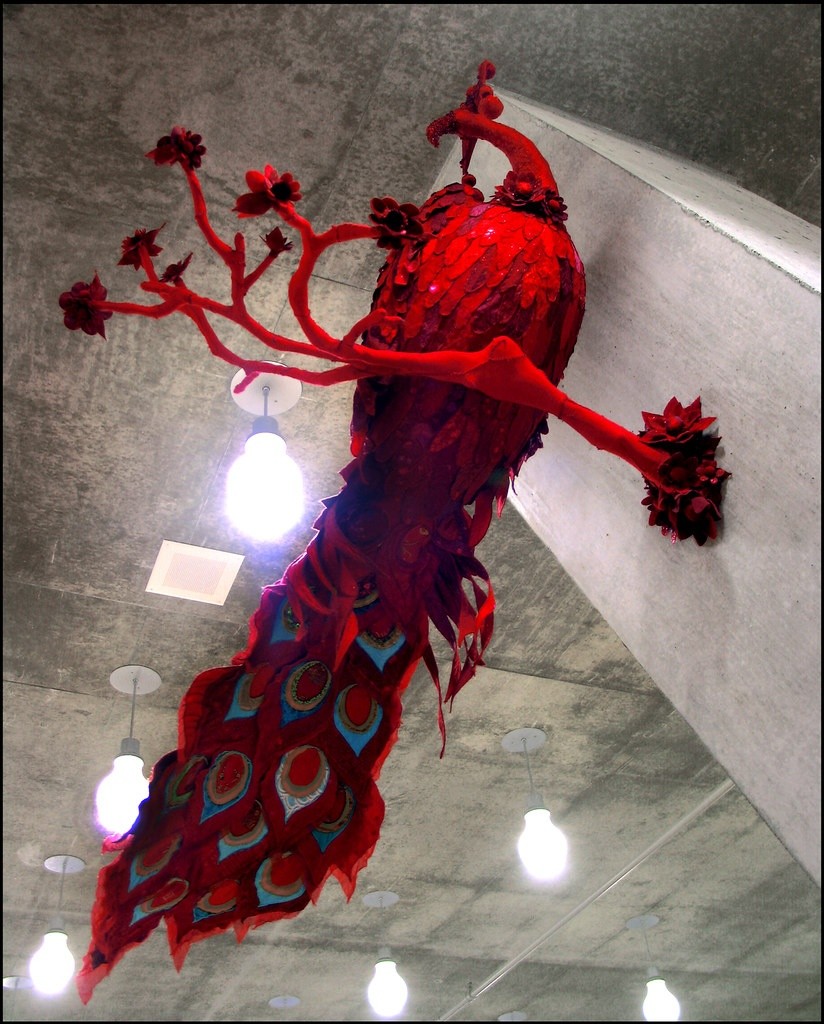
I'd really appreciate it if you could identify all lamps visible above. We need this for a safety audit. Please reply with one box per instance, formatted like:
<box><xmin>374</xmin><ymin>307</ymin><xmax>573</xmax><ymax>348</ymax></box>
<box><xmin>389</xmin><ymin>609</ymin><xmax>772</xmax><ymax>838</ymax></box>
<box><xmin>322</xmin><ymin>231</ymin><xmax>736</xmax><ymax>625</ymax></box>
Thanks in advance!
<box><xmin>28</xmin><ymin>854</ymin><xmax>88</xmax><ymax>997</ymax></box>
<box><xmin>626</xmin><ymin>914</ymin><xmax>681</xmax><ymax>1021</ymax></box>
<box><xmin>501</xmin><ymin>727</ymin><xmax>569</xmax><ymax>881</ymax></box>
<box><xmin>363</xmin><ymin>890</ymin><xmax>408</xmax><ymax>1018</ymax></box>
<box><xmin>223</xmin><ymin>360</ymin><xmax>307</xmax><ymax>546</ymax></box>
<box><xmin>92</xmin><ymin>664</ymin><xmax>163</xmax><ymax>836</ymax></box>
